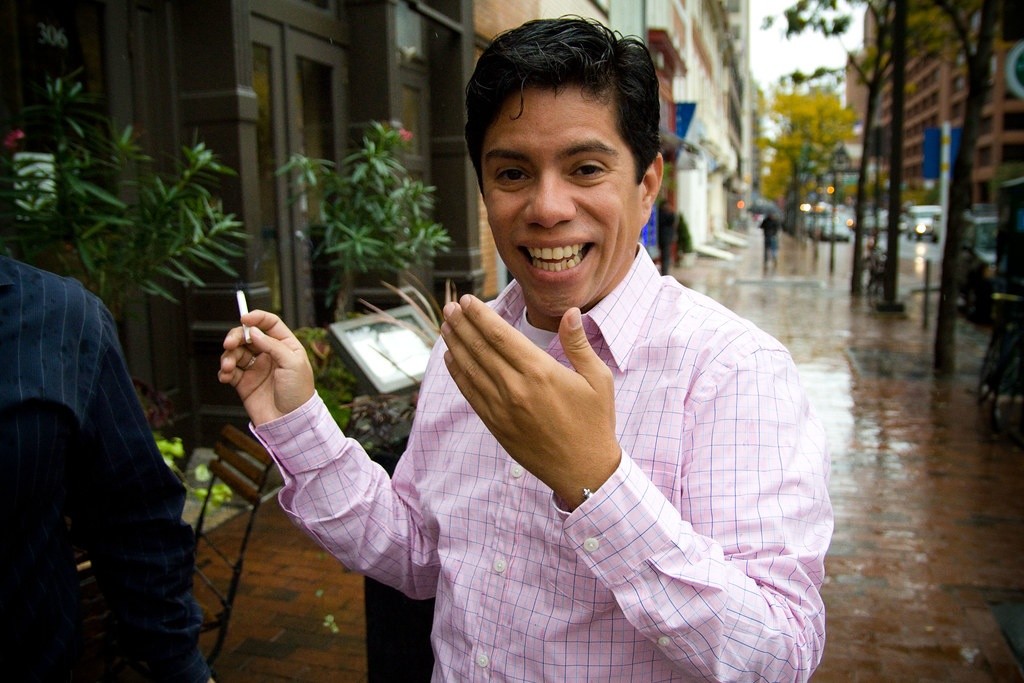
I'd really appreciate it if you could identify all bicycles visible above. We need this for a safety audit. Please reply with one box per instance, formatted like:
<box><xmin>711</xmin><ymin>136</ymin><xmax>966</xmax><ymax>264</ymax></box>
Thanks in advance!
<box><xmin>976</xmin><ymin>295</ymin><xmax>1024</xmax><ymax>449</ymax></box>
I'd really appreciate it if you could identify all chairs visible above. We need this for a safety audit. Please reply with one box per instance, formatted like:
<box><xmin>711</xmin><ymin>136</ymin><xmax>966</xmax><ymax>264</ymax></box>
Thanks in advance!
<box><xmin>98</xmin><ymin>424</ymin><xmax>275</xmax><ymax>683</ymax></box>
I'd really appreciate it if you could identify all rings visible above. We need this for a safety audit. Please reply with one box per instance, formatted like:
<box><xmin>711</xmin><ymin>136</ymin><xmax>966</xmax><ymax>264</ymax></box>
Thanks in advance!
<box><xmin>242</xmin><ymin>354</ymin><xmax>257</xmax><ymax>370</ymax></box>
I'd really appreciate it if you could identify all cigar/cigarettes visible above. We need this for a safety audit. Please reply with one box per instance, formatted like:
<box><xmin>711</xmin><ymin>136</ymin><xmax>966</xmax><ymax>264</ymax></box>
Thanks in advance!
<box><xmin>236</xmin><ymin>290</ymin><xmax>253</xmax><ymax>343</ymax></box>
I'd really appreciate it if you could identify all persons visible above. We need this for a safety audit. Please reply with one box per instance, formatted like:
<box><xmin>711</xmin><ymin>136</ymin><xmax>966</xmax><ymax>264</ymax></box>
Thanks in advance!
<box><xmin>758</xmin><ymin>213</ymin><xmax>782</xmax><ymax>277</ymax></box>
<box><xmin>657</xmin><ymin>200</ymin><xmax>693</xmax><ymax>276</ymax></box>
<box><xmin>0</xmin><ymin>249</ymin><xmax>215</xmax><ymax>683</ymax></box>
<box><xmin>220</xmin><ymin>14</ymin><xmax>835</xmax><ymax>683</ymax></box>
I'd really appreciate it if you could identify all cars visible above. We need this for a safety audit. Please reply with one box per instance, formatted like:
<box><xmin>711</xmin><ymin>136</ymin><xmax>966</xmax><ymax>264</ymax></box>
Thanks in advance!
<box><xmin>751</xmin><ymin>197</ymin><xmax>999</xmax><ymax>282</ymax></box>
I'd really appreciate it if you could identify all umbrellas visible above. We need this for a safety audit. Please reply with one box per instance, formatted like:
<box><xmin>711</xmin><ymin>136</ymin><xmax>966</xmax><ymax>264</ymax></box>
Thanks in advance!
<box><xmin>747</xmin><ymin>200</ymin><xmax>780</xmax><ymax>220</ymax></box>
